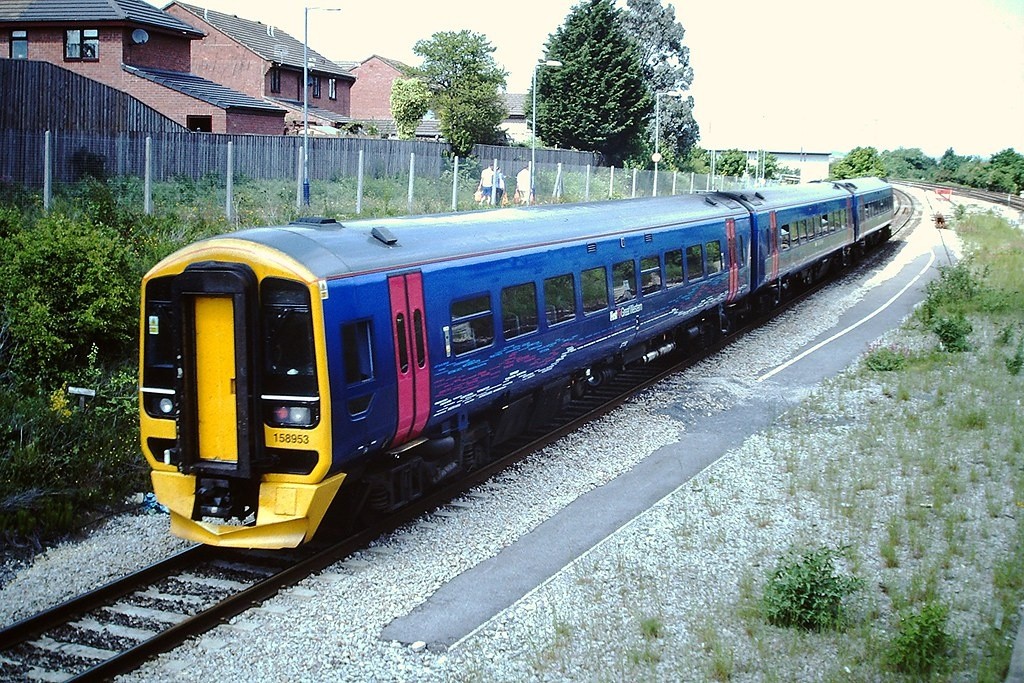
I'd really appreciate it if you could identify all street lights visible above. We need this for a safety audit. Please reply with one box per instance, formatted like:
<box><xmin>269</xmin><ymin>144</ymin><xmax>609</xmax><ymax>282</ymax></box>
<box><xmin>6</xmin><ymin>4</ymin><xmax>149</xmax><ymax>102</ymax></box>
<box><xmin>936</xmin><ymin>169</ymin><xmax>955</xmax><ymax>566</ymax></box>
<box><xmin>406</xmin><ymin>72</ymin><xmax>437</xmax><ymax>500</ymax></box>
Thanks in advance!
<box><xmin>303</xmin><ymin>7</ymin><xmax>341</xmax><ymax>206</ymax></box>
<box><xmin>653</xmin><ymin>91</ymin><xmax>681</xmax><ymax>195</ymax></box>
<box><xmin>530</xmin><ymin>61</ymin><xmax>563</xmax><ymax>202</ymax></box>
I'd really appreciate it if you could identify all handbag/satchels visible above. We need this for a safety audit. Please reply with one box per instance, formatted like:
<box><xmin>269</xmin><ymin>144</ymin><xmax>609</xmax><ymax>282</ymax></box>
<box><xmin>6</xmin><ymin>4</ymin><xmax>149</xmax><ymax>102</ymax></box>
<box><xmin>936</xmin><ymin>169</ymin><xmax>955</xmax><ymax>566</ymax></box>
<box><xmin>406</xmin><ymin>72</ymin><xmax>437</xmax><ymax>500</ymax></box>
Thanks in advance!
<box><xmin>502</xmin><ymin>192</ymin><xmax>507</xmax><ymax>204</ymax></box>
<box><xmin>514</xmin><ymin>189</ymin><xmax>519</xmax><ymax>204</ymax></box>
<box><xmin>475</xmin><ymin>189</ymin><xmax>482</xmax><ymax>202</ymax></box>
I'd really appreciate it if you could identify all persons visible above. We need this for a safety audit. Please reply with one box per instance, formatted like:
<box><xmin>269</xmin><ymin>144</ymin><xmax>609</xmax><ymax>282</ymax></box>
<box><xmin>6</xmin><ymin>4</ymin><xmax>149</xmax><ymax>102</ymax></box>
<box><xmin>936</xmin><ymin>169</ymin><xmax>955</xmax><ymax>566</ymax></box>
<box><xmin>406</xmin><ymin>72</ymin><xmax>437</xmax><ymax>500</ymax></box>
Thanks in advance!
<box><xmin>492</xmin><ymin>166</ymin><xmax>506</xmax><ymax>204</ymax></box>
<box><xmin>477</xmin><ymin>161</ymin><xmax>494</xmax><ymax>208</ymax></box>
<box><xmin>515</xmin><ymin>162</ymin><xmax>529</xmax><ymax>205</ymax></box>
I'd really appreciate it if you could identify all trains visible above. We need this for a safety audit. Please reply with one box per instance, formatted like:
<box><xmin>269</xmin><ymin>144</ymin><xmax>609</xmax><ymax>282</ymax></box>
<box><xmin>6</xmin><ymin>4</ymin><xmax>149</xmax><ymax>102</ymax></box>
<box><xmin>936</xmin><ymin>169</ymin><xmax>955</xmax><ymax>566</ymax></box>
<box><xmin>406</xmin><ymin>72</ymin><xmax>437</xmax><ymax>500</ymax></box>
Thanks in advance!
<box><xmin>140</xmin><ymin>177</ymin><xmax>894</xmax><ymax>567</ymax></box>
